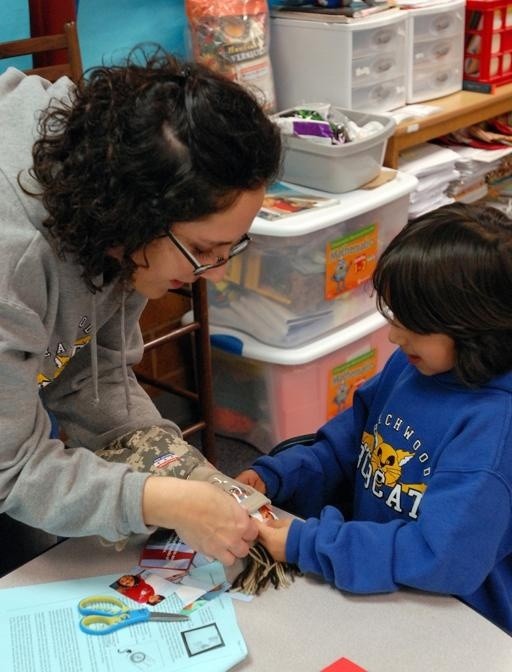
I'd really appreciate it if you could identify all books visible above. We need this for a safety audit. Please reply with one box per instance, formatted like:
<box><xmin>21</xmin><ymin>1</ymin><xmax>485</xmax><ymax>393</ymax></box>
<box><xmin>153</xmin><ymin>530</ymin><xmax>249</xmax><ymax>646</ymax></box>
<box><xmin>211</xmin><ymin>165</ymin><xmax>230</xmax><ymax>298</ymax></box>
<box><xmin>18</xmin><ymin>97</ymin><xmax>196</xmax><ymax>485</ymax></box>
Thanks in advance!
<box><xmin>270</xmin><ymin>0</ymin><xmax>399</xmax><ymax>24</ymax></box>
<box><xmin>206</xmin><ymin>280</ymin><xmax>335</xmax><ymax>345</ymax></box>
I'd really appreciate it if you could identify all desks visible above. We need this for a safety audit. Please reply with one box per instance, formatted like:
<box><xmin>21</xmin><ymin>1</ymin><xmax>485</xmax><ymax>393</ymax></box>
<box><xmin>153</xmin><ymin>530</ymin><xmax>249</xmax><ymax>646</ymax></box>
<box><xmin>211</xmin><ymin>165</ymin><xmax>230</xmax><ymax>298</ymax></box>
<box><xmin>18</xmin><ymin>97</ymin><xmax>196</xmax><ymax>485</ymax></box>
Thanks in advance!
<box><xmin>0</xmin><ymin>505</ymin><xmax>511</xmax><ymax>672</ymax></box>
<box><xmin>383</xmin><ymin>82</ymin><xmax>511</xmax><ymax>171</ymax></box>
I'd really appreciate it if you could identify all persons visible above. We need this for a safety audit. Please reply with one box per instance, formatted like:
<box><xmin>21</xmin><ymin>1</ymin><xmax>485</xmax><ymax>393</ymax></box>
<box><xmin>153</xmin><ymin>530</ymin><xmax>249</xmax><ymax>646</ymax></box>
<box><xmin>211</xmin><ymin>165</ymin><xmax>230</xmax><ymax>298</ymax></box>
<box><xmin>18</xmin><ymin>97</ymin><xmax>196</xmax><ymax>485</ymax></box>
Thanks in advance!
<box><xmin>0</xmin><ymin>41</ymin><xmax>282</xmax><ymax>567</ymax></box>
<box><xmin>116</xmin><ymin>576</ymin><xmax>165</xmax><ymax>604</ymax></box>
<box><xmin>236</xmin><ymin>201</ymin><xmax>511</xmax><ymax>636</ymax></box>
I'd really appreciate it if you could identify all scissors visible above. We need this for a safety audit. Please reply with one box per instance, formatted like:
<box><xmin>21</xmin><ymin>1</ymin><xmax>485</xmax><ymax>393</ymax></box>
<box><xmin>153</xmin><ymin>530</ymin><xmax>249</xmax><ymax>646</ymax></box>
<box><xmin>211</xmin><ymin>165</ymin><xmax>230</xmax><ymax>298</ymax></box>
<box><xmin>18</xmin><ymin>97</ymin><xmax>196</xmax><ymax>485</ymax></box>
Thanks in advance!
<box><xmin>79</xmin><ymin>596</ymin><xmax>188</xmax><ymax>635</ymax></box>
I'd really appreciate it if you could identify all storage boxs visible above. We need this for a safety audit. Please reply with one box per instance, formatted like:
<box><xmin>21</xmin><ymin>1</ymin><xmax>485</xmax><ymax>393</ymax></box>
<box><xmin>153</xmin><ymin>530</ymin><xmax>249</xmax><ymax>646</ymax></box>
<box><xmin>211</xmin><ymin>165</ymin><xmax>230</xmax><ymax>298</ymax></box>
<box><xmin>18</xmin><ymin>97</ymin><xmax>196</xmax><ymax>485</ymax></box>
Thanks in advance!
<box><xmin>408</xmin><ymin>0</ymin><xmax>467</xmax><ymax>104</ymax></box>
<box><xmin>269</xmin><ymin>104</ymin><xmax>396</xmax><ymax>192</ymax></box>
<box><xmin>207</xmin><ymin>167</ymin><xmax>419</xmax><ymax>347</ymax></box>
<box><xmin>181</xmin><ymin>306</ymin><xmax>401</xmax><ymax>455</ymax></box>
<box><xmin>269</xmin><ymin>11</ymin><xmax>409</xmax><ymax>113</ymax></box>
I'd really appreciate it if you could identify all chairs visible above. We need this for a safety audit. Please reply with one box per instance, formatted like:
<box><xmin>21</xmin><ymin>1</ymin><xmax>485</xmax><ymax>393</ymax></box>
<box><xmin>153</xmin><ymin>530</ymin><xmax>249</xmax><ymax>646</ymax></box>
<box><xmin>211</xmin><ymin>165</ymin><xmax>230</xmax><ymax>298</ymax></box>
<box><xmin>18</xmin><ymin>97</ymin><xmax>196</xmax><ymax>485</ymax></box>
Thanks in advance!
<box><xmin>0</xmin><ymin>21</ymin><xmax>216</xmax><ymax>468</ymax></box>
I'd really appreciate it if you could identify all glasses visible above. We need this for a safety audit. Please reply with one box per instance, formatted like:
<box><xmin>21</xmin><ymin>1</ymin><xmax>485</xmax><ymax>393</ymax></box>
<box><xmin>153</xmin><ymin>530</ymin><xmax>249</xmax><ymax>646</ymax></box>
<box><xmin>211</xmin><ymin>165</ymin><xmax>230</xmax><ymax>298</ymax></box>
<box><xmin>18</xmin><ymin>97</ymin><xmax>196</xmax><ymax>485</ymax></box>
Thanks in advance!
<box><xmin>163</xmin><ymin>229</ymin><xmax>253</xmax><ymax>276</ymax></box>
